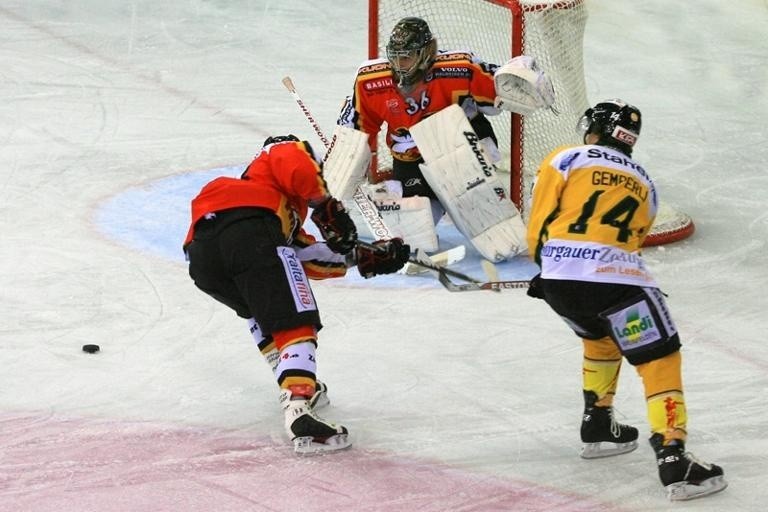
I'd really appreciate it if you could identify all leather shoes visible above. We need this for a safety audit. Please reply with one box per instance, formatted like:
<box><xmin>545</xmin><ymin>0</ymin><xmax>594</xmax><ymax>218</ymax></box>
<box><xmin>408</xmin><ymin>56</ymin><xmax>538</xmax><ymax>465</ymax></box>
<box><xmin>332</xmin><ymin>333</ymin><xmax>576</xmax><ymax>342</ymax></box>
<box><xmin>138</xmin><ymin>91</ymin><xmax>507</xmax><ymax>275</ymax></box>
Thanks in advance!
<box><xmin>357</xmin><ymin>236</ymin><xmax>411</xmax><ymax>280</ymax></box>
<box><xmin>310</xmin><ymin>195</ymin><xmax>357</xmax><ymax>255</ymax></box>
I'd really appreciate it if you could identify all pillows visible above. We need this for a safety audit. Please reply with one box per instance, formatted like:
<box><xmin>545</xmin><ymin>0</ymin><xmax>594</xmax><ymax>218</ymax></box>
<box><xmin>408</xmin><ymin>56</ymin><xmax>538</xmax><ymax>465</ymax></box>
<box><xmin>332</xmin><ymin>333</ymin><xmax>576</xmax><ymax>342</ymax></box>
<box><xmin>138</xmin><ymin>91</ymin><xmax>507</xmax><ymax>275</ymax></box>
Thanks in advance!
<box><xmin>385</xmin><ymin>17</ymin><xmax>439</xmax><ymax>53</ymax></box>
<box><xmin>584</xmin><ymin>98</ymin><xmax>641</xmax><ymax>156</ymax></box>
<box><xmin>263</xmin><ymin>134</ymin><xmax>315</xmax><ymax>161</ymax></box>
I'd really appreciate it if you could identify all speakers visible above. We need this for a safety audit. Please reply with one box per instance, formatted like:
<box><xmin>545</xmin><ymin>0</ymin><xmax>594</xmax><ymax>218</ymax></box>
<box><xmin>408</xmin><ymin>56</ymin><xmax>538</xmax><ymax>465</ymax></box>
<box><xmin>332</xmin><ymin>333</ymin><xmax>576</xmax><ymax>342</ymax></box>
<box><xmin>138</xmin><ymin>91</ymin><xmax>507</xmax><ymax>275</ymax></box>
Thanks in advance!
<box><xmin>312</xmin><ymin>382</ymin><xmax>329</xmax><ymax>394</ymax></box>
<box><xmin>580</xmin><ymin>389</ymin><xmax>639</xmax><ymax>444</ymax></box>
<box><xmin>278</xmin><ymin>390</ymin><xmax>350</xmax><ymax>441</ymax></box>
<box><xmin>649</xmin><ymin>433</ymin><xmax>723</xmax><ymax>487</ymax></box>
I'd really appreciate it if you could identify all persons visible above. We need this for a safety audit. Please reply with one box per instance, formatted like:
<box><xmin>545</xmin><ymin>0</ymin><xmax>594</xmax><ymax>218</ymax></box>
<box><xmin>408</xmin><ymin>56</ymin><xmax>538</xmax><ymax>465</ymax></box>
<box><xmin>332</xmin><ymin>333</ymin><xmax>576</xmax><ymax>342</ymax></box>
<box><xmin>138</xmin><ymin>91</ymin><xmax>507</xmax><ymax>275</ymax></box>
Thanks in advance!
<box><xmin>183</xmin><ymin>135</ymin><xmax>410</xmax><ymax>454</ymax></box>
<box><xmin>525</xmin><ymin>98</ymin><xmax>726</xmax><ymax>499</ymax></box>
<box><xmin>336</xmin><ymin>16</ymin><xmax>502</xmax><ymax>228</ymax></box>
